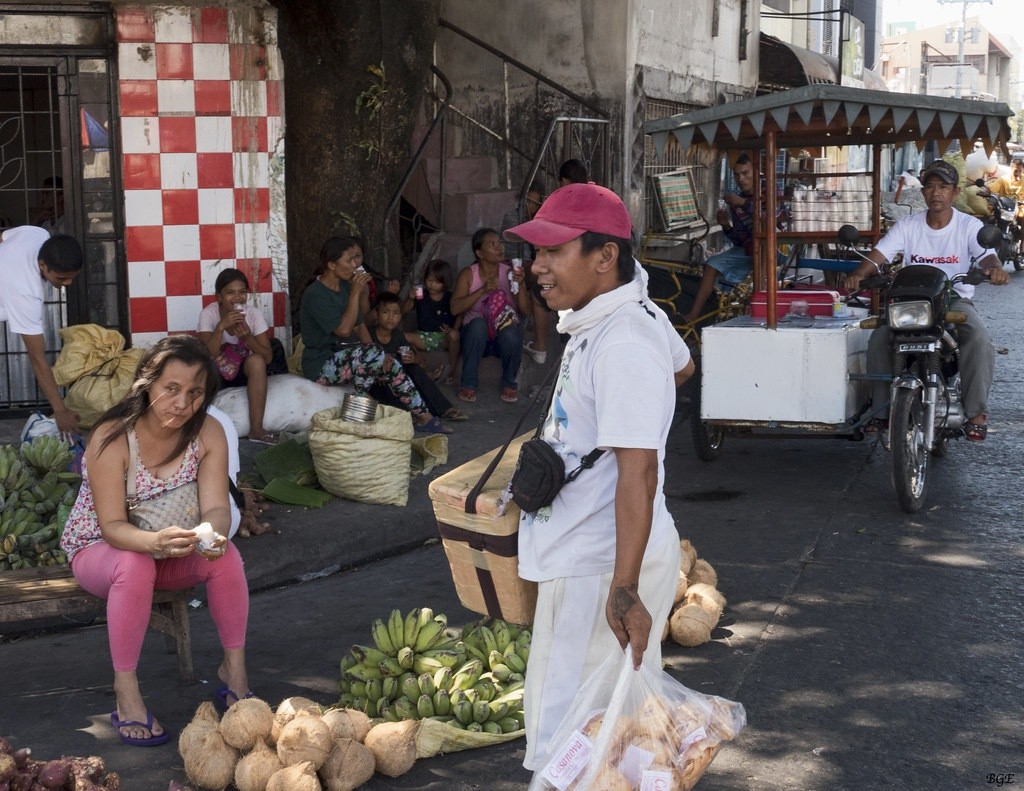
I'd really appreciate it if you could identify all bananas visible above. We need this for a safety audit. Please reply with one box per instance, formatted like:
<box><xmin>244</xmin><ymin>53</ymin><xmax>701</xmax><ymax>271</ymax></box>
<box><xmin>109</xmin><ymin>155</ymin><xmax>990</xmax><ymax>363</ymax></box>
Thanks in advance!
<box><xmin>330</xmin><ymin>606</ymin><xmax>533</xmax><ymax>734</ymax></box>
<box><xmin>0</xmin><ymin>432</ymin><xmax>84</xmax><ymax>572</ymax></box>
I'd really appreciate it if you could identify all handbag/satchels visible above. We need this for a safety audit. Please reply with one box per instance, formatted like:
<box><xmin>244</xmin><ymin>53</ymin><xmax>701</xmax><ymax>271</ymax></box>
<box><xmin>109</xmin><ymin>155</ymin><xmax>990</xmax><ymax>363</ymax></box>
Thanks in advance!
<box><xmin>528</xmin><ymin>643</ymin><xmax>748</xmax><ymax>791</ymax></box>
<box><xmin>507</xmin><ymin>440</ymin><xmax>566</xmax><ymax>512</ymax></box>
<box><xmin>130</xmin><ymin>479</ymin><xmax>201</xmax><ymax>561</ymax></box>
<box><xmin>481</xmin><ymin>290</ymin><xmax>521</xmax><ymax>339</ymax></box>
<box><xmin>330</xmin><ymin>338</ymin><xmax>362</xmax><ymax>352</ymax></box>
<box><xmin>213</xmin><ymin>342</ymin><xmax>250</xmax><ymax>380</ymax></box>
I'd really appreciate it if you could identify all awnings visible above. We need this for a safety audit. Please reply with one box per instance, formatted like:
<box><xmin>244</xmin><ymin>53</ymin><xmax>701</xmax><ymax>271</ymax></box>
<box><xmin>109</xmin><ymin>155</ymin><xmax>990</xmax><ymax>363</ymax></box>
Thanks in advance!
<box><xmin>758</xmin><ymin>33</ymin><xmax>889</xmax><ymax>96</ymax></box>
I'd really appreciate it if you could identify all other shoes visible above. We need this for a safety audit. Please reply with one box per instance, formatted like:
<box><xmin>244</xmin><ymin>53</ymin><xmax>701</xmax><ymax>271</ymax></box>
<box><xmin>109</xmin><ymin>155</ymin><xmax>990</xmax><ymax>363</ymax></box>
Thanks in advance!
<box><xmin>668</xmin><ymin>312</ymin><xmax>694</xmax><ymax>334</ymax></box>
<box><xmin>521</xmin><ymin>339</ymin><xmax>547</xmax><ymax>364</ymax></box>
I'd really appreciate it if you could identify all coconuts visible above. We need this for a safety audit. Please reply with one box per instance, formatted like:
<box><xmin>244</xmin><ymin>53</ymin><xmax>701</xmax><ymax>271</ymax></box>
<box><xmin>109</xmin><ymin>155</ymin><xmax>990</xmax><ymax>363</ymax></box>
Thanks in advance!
<box><xmin>178</xmin><ymin>697</ymin><xmax>421</xmax><ymax>791</ymax></box>
<box><xmin>661</xmin><ymin>539</ymin><xmax>725</xmax><ymax>646</ymax></box>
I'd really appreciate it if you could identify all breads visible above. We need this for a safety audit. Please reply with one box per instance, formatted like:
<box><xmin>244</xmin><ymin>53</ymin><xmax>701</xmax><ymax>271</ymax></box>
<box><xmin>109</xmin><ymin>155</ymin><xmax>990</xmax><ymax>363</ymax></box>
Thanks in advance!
<box><xmin>558</xmin><ymin>692</ymin><xmax>736</xmax><ymax>791</ymax></box>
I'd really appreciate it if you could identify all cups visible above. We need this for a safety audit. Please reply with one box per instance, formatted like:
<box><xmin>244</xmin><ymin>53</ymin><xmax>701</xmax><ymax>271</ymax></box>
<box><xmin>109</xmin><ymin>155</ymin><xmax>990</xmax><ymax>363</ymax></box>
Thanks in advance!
<box><xmin>234</xmin><ymin>304</ymin><xmax>245</xmax><ymax>315</ymax></box>
<box><xmin>792</xmin><ymin>173</ymin><xmax>871</xmax><ymax>231</ymax></box>
<box><xmin>511</xmin><ymin>258</ymin><xmax>522</xmax><ymax>272</ymax></box>
<box><xmin>353</xmin><ymin>265</ymin><xmax>366</xmax><ymax>276</ymax></box>
<box><xmin>414</xmin><ymin>285</ymin><xmax>423</xmax><ymax>299</ymax></box>
<box><xmin>400</xmin><ymin>346</ymin><xmax>409</xmax><ymax>358</ymax></box>
<box><xmin>719</xmin><ymin>199</ymin><xmax>727</xmax><ymax>210</ymax></box>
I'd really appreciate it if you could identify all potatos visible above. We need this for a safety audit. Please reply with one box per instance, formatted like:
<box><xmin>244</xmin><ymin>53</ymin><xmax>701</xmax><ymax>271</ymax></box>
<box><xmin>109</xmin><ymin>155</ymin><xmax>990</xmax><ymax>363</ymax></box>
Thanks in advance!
<box><xmin>236</xmin><ymin>479</ymin><xmax>272</xmax><ymax>537</ymax></box>
<box><xmin>0</xmin><ymin>737</ymin><xmax>120</xmax><ymax>791</ymax></box>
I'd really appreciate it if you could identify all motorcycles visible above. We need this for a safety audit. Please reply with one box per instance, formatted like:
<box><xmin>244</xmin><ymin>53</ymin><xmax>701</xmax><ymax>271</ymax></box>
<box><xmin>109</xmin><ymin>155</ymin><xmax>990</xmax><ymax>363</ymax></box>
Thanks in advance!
<box><xmin>975</xmin><ymin>178</ymin><xmax>1024</xmax><ymax>271</ymax></box>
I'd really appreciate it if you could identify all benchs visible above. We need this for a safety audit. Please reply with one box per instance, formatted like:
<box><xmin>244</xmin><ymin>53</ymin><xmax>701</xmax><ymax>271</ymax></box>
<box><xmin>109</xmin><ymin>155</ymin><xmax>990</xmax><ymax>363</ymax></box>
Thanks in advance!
<box><xmin>0</xmin><ymin>563</ymin><xmax>198</xmax><ymax>693</ymax></box>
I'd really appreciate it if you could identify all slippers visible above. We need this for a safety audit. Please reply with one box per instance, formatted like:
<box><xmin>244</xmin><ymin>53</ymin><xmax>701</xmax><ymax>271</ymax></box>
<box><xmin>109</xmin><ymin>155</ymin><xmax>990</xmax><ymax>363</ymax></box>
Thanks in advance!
<box><xmin>864</xmin><ymin>417</ymin><xmax>889</xmax><ymax>435</ymax></box>
<box><xmin>439</xmin><ymin>407</ymin><xmax>468</xmax><ymax>420</ymax></box>
<box><xmin>457</xmin><ymin>385</ymin><xmax>478</xmax><ymax>402</ymax></box>
<box><xmin>499</xmin><ymin>384</ymin><xmax>519</xmax><ymax>402</ymax></box>
<box><xmin>964</xmin><ymin>420</ymin><xmax>988</xmax><ymax>442</ymax></box>
<box><xmin>216</xmin><ymin>687</ymin><xmax>254</xmax><ymax>711</ymax></box>
<box><xmin>249</xmin><ymin>431</ymin><xmax>282</xmax><ymax>445</ymax></box>
<box><xmin>414</xmin><ymin>418</ymin><xmax>451</xmax><ymax>434</ymax></box>
<box><xmin>111</xmin><ymin>707</ymin><xmax>169</xmax><ymax>746</ymax></box>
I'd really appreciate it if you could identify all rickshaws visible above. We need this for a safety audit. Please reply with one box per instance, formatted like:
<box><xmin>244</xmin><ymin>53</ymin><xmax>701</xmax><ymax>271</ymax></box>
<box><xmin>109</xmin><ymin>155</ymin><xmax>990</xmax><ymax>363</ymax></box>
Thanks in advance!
<box><xmin>645</xmin><ymin>82</ymin><xmax>1004</xmax><ymax>514</ymax></box>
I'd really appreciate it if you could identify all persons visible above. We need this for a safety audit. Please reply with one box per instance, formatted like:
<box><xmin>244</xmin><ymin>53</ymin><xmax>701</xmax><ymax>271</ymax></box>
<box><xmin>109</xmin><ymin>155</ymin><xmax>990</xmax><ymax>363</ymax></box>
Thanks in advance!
<box><xmin>977</xmin><ymin>159</ymin><xmax>1024</xmax><ymax>262</ymax></box>
<box><xmin>908</xmin><ymin>169</ymin><xmax>925</xmax><ymax>186</ymax></box>
<box><xmin>452</xmin><ymin>227</ymin><xmax>531</xmax><ymax>402</ymax></box>
<box><xmin>200</xmin><ymin>268</ymin><xmax>280</xmax><ymax>445</ymax></box>
<box><xmin>0</xmin><ymin>225</ymin><xmax>84</xmax><ymax>444</ymax></box>
<box><xmin>522</xmin><ymin>183</ymin><xmax>552</xmax><ymax>364</ymax></box>
<box><xmin>682</xmin><ymin>153</ymin><xmax>789</xmax><ymax>334</ymax></box>
<box><xmin>560</xmin><ymin>159</ymin><xmax>589</xmax><ymax>185</ymax></box>
<box><xmin>59</xmin><ymin>336</ymin><xmax>255</xmax><ymax>747</ymax></box>
<box><xmin>845</xmin><ymin>160</ymin><xmax>1009</xmax><ymax>441</ymax></box>
<box><xmin>298</xmin><ymin>235</ymin><xmax>468</xmax><ymax>434</ymax></box>
<box><xmin>501</xmin><ymin>180</ymin><xmax>694</xmax><ymax>770</ymax></box>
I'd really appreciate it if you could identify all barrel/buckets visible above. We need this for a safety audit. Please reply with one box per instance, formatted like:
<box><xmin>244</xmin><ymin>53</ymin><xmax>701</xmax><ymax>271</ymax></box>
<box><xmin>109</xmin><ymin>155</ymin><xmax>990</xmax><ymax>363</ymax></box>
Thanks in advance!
<box><xmin>340</xmin><ymin>393</ymin><xmax>378</xmax><ymax>422</ymax></box>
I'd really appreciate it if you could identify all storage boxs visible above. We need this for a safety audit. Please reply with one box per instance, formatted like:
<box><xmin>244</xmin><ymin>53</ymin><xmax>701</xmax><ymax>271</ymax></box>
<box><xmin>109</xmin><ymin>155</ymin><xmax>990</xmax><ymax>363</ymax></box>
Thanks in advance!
<box><xmin>427</xmin><ymin>429</ymin><xmax>547</xmax><ymax>628</ymax></box>
<box><xmin>700</xmin><ymin>282</ymin><xmax>880</xmax><ymax>425</ymax></box>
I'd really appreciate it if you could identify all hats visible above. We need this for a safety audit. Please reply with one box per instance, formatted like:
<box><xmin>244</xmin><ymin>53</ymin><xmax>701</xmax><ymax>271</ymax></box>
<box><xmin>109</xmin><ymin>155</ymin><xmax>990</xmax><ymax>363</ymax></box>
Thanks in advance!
<box><xmin>503</xmin><ymin>181</ymin><xmax>632</xmax><ymax>247</ymax></box>
<box><xmin>922</xmin><ymin>160</ymin><xmax>959</xmax><ymax>190</ymax></box>
